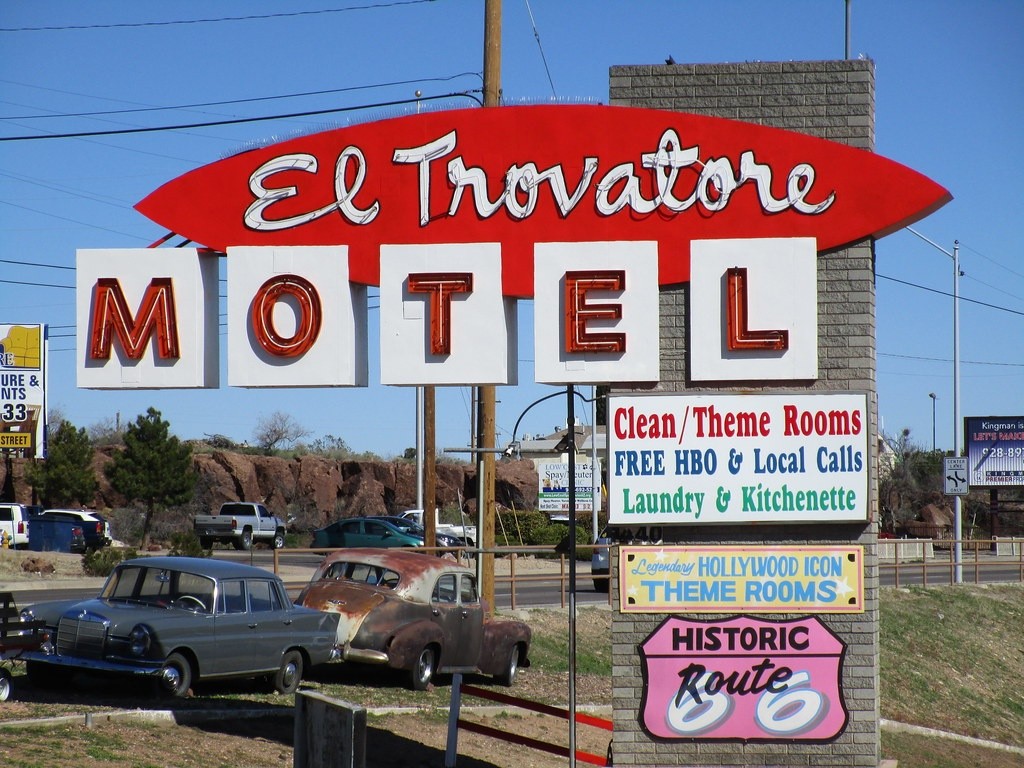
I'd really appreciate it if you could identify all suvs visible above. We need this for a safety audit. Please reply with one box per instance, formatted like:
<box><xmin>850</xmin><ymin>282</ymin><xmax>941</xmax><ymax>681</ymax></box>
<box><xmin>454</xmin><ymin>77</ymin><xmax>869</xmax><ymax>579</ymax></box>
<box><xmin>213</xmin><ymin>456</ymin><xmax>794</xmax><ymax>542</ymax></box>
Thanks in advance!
<box><xmin>0</xmin><ymin>500</ymin><xmax>113</xmax><ymax>554</ymax></box>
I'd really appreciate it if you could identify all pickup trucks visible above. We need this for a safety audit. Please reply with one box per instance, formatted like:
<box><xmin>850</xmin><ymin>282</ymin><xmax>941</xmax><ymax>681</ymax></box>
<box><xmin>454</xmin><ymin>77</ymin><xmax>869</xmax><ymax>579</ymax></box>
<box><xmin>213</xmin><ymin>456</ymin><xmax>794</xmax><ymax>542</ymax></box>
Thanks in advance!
<box><xmin>397</xmin><ymin>507</ymin><xmax>477</xmax><ymax>559</ymax></box>
<box><xmin>193</xmin><ymin>502</ymin><xmax>288</xmax><ymax>551</ymax></box>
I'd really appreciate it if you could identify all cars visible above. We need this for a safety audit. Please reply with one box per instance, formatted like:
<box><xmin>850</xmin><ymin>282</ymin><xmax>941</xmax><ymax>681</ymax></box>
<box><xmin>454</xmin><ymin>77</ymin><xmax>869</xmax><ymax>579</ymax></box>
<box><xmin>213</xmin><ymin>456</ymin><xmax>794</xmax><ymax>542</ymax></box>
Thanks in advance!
<box><xmin>295</xmin><ymin>547</ymin><xmax>532</xmax><ymax>689</ymax></box>
<box><xmin>365</xmin><ymin>514</ymin><xmax>464</xmax><ymax>558</ymax></box>
<box><xmin>592</xmin><ymin>526</ymin><xmax>612</xmax><ymax>592</ymax></box>
<box><xmin>310</xmin><ymin>517</ymin><xmax>425</xmax><ymax>557</ymax></box>
<box><xmin>14</xmin><ymin>556</ymin><xmax>342</xmax><ymax>703</ymax></box>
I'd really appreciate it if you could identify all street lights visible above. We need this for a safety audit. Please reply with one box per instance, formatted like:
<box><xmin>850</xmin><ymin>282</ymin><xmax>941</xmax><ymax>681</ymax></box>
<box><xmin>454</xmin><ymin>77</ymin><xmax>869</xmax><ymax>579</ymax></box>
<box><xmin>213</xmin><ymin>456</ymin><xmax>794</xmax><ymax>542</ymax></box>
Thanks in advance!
<box><xmin>929</xmin><ymin>392</ymin><xmax>936</xmax><ymax>451</ymax></box>
